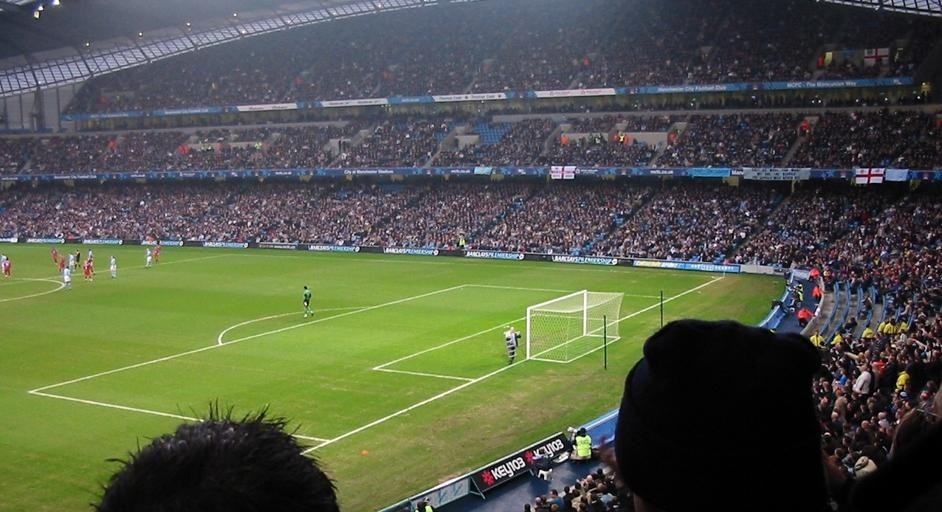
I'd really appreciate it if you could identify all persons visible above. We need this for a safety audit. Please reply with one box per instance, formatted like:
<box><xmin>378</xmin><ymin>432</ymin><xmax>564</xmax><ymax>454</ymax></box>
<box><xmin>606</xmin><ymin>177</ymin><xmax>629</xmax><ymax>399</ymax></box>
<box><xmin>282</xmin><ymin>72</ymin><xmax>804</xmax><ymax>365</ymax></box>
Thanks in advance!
<box><xmin>0</xmin><ymin>0</ymin><xmax>941</xmax><ymax>273</ymax></box>
<box><xmin>91</xmin><ymin>397</ymin><xmax>341</xmax><ymax>512</ymax></box>
<box><xmin>303</xmin><ymin>285</ymin><xmax>315</xmax><ymax>319</ymax></box>
<box><xmin>517</xmin><ymin>434</ymin><xmax>632</xmax><ymax>511</ymax></box>
<box><xmin>755</xmin><ymin>273</ymin><xmax>941</xmax><ymax>512</ymax></box>
<box><xmin>503</xmin><ymin>326</ymin><xmax>521</xmax><ymax>365</ymax></box>
<box><xmin>109</xmin><ymin>255</ymin><xmax>118</xmax><ymax>279</ymax></box>
<box><xmin>83</xmin><ymin>259</ymin><xmax>94</xmax><ymax>283</ymax></box>
<box><xmin>570</xmin><ymin>429</ymin><xmax>593</xmax><ymax>460</ymax></box>
<box><xmin>63</xmin><ymin>266</ymin><xmax>73</xmax><ymax>291</ymax></box>
<box><xmin>4</xmin><ymin>257</ymin><xmax>12</xmax><ymax>278</ymax></box>
<box><xmin>414</xmin><ymin>500</ymin><xmax>436</xmax><ymax>512</ymax></box>
